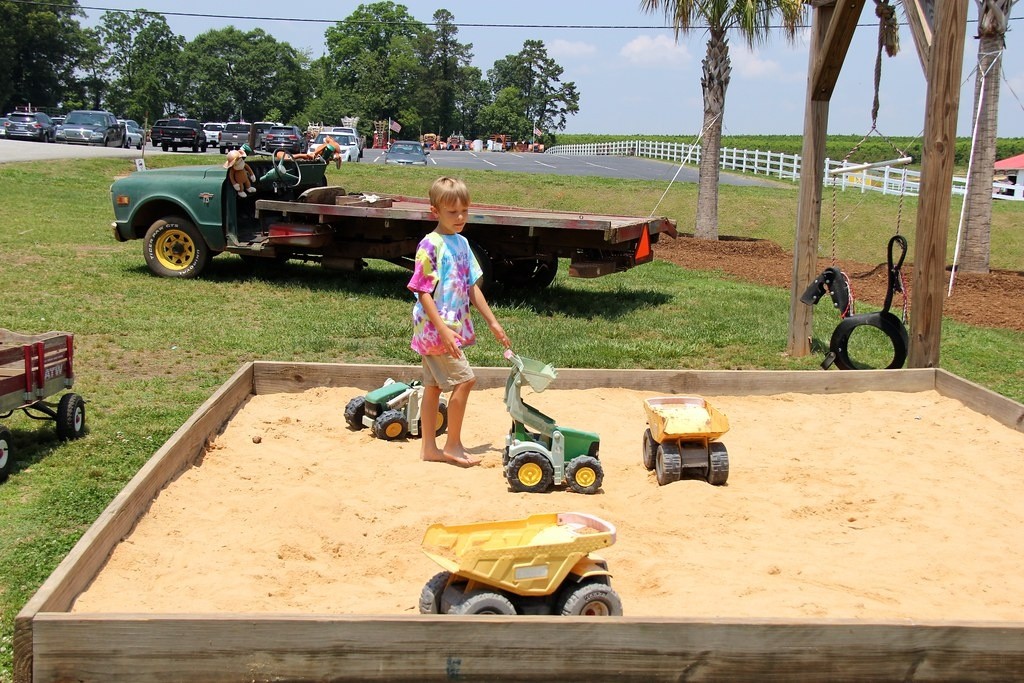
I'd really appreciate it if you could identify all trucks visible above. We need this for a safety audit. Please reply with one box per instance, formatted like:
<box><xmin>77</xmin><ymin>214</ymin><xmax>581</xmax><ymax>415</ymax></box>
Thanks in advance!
<box><xmin>111</xmin><ymin>144</ymin><xmax>679</xmax><ymax>302</ymax></box>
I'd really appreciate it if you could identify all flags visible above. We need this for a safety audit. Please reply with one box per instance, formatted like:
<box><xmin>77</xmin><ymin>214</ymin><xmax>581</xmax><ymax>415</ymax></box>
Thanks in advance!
<box><xmin>390</xmin><ymin>120</ymin><xmax>402</xmax><ymax>133</ymax></box>
<box><xmin>534</xmin><ymin>127</ymin><xmax>541</xmax><ymax>136</ymax></box>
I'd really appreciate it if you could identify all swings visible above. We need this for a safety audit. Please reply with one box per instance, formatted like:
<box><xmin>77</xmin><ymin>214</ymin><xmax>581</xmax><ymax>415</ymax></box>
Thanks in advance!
<box><xmin>799</xmin><ymin>0</ymin><xmax>909</xmax><ymax>370</ymax></box>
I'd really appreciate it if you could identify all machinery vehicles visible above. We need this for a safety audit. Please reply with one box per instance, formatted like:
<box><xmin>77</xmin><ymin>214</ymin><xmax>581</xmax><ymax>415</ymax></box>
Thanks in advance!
<box><xmin>344</xmin><ymin>378</ymin><xmax>449</xmax><ymax>443</ymax></box>
<box><xmin>641</xmin><ymin>395</ymin><xmax>731</xmax><ymax>486</ymax></box>
<box><xmin>419</xmin><ymin>511</ymin><xmax>625</xmax><ymax>617</ymax></box>
<box><xmin>501</xmin><ymin>350</ymin><xmax>605</xmax><ymax>494</ymax></box>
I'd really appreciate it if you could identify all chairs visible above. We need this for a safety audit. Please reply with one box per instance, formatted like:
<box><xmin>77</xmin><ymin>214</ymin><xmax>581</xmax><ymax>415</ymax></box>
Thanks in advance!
<box><xmin>337</xmin><ymin>137</ymin><xmax>346</xmax><ymax>145</ymax></box>
<box><xmin>268</xmin><ymin>186</ymin><xmax>346</xmax><ymax>244</ymax></box>
<box><xmin>396</xmin><ymin>148</ymin><xmax>405</xmax><ymax>153</ymax></box>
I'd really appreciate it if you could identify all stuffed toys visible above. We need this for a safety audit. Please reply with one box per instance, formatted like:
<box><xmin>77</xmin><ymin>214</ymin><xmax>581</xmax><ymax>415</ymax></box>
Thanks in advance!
<box><xmin>223</xmin><ymin>150</ymin><xmax>257</xmax><ymax>197</ymax></box>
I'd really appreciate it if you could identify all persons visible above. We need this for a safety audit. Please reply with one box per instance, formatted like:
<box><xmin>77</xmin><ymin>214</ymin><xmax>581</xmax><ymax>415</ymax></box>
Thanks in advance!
<box><xmin>406</xmin><ymin>177</ymin><xmax>510</xmax><ymax>465</ymax></box>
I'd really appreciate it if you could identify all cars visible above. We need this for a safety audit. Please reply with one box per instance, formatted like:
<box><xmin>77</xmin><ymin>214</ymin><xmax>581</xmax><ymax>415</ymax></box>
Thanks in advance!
<box><xmin>151</xmin><ymin>117</ymin><xmax>365</xmax><ymax>163</ymax></box>
<box><xmin>384</xmin><ymin>140</ymin><xmax>431</xmax><ymax>166</ymax></box>
<box><xmin>0</xmin><ymin>110</ymin><xmax>145</xmax><ymax>150</ymax></box>
